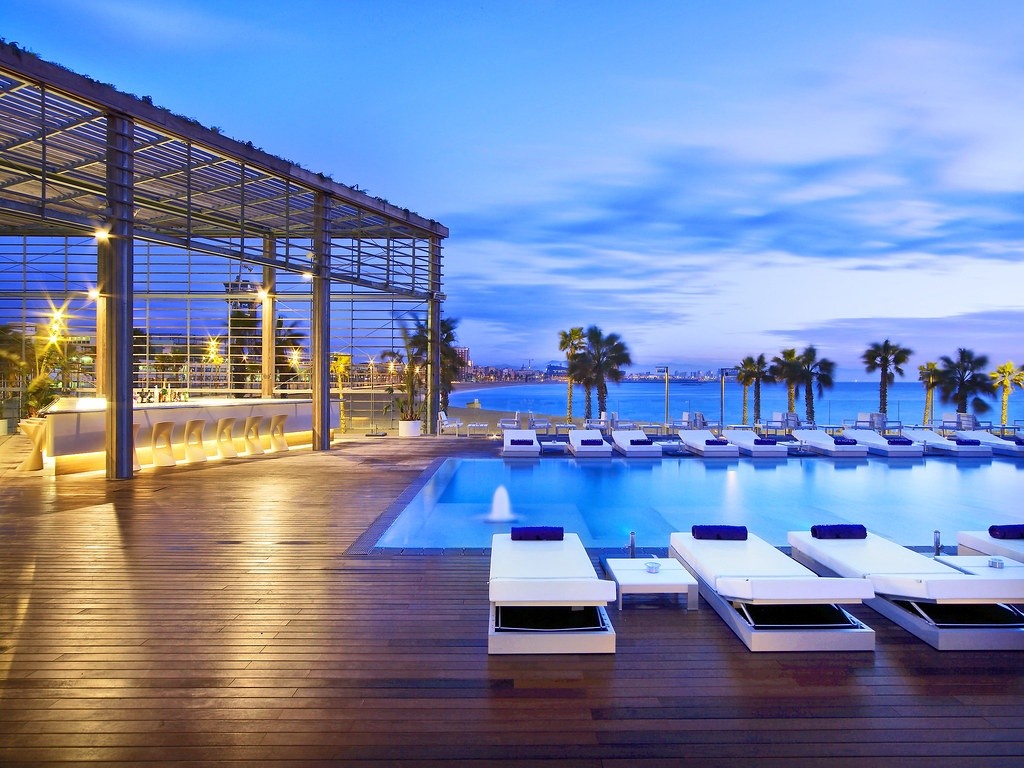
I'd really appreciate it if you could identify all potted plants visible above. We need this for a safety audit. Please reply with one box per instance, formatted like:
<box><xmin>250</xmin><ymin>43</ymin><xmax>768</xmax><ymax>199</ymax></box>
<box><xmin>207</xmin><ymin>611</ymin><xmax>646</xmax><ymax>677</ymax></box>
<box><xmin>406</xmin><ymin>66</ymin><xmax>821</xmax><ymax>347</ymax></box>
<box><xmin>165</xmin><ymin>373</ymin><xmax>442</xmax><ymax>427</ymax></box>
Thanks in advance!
<box><xmin>381</xmin><ymin>311</ymin><xmax>433</xmax><ymax>437</ymax></box>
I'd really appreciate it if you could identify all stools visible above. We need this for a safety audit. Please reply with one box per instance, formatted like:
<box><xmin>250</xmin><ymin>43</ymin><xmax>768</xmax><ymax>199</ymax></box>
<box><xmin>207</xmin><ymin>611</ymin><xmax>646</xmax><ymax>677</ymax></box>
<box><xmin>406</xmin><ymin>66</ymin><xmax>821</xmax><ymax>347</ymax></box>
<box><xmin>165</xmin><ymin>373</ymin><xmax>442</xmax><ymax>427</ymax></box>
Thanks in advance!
<box><xmin>243</xmin><ymin>414</ymin><xmax>265</xmax><ymax>455</ymax></box>
<box><xmin>216</xmin><ymin>416</ymin><xmax>238</xmax><ymax>459</ymax></box>
<box><xmin>131</xmin><ymin>421</ymin><xmax>143</xmax><ymax>471</ymax></box>
<box><xmin>13</xmin><ymin>416</ymin><xmax>46</xmax><ymax>472</ymax></box>
<box><xmin>151</xmin><ymin>418</ymin><xmax>176</xmax><ymax>467</ymax></box>
<box><xmin>184</xmin><ymin>419</ymin><xmax>207</xmax><ymax>463</ymax></box>
<box><xmin>269</xmin><ymin>412</ymin><xmax>289</xmax><ymax>453</ymax></box>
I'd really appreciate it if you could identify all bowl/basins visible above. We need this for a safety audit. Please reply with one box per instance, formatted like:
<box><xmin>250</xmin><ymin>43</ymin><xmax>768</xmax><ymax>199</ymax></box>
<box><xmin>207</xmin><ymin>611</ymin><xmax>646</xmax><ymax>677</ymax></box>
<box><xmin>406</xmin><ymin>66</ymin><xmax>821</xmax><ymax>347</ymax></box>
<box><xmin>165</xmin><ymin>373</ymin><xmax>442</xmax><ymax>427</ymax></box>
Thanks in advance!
<box><xmin>644</xmin><ymin>561</ymin><xmax>662</xmax><ymax>574</ymax></box>
<box><xmin>987</xmin><ymin>557</ymin><xmax>1005</xmax><ymax>569</ymax></box>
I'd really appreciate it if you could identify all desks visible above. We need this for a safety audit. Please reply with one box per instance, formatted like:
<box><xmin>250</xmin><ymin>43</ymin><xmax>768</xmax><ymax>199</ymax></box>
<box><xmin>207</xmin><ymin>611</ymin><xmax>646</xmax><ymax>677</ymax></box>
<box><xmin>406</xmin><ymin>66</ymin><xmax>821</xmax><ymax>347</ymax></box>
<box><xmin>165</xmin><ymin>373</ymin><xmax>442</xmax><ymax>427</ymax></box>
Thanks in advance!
<box><xmin>655</xmin><ymin>441</ymin><xmax>685</xmax><ymax>453</ymax></box>
<box><xmin>466</xmin><ymin>423</ymin><xmax>489</xmax><ymax>437</ymax></box>
<box><xmin>913</xmin><ymin>441</ymin><xmax>932</xmax><ymax>451</ymax></box>
<box><xmin>777</xmin><ymin>441</ymin><xmax>810</xmax><ymax>452</ymax></box>
<box><xmin>993</xmin><ymin>424</ymin><xmax>1020</xmax><ymax>436</ymax></box>
<box><xmin>934</xmin><ymin>555</ymin><xmax>1024</xmax><ymax>578</ymax></box>
<box><xmin>541</xmin><ymin>441</ymin><xmax>567</xmax><ymax>453</ymax></box>
<box><xmin>726</xmin><ymin>425</ymin><xmax>753</xmax><ymax>431</ymax></box>
<box><xmin>604</xmin><ymin>555</ymin><xmax>699</xmax><ymax>612</ymax></box>
<box><xmin>903</xmin><ymin>425</ymin><xmax>934</xmax><ymax>431</ymax></box>
<box><xmin>40</xmin><ymin>394</ymin><xmax>348</xmax><ymax>472</ymax></box>
<box><xmin>556</xmin><ymin>423</ymin><xmax>577</xmax><ymax>438</ymax></box>
<box><xmin>639</xmin><ymin>424</ymin><xmax>662</xmax><ymax>437</ymax></box>
<box><xmin>818</xmin><ymin>425</ymin><xmax>845</xmax><ymax>435</ymax></box>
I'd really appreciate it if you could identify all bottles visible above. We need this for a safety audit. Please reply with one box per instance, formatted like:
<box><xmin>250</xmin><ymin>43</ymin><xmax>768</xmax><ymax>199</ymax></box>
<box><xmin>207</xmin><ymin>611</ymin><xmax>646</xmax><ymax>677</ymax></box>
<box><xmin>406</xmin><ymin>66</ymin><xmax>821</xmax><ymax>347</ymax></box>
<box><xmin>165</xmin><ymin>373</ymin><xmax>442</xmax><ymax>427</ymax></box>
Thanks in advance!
<box><xmin>134</xmin><ymin>380</ymin><xmax>190</xmax><ymax>402</ymax></box>
<box><xmin>61</xmin><ymin>387</ymin><xmax>76</xmax><ymax>395</ymax></box>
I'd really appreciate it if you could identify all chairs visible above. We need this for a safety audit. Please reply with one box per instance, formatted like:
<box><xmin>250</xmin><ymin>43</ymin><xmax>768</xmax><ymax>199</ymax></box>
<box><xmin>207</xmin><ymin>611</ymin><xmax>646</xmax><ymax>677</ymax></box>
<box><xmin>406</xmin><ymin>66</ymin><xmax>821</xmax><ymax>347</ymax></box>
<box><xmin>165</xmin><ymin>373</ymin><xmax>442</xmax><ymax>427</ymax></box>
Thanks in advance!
<box><xmin>431</xmin><ymin>400</ymin><xmax>1024</xmax><ymax>462</ymax></box>
<box><xmin>957</xmin><ymin>523</ymin><xmax>1024</xmax><ymax>564</ymax></box>
<box><xmin>786</xmin><ymin>523</ymin><xmax>1024</xmax><ymax>651</ymax></box>
<box><xmin>485</xmin><ymin>526</ymin><xmax>618</xmax><ymax>655</ymax></box>
<box><xmin>667</xmin><ymin>525</ymin><xmax>876</xmax><ymax>656</ymax></box>
